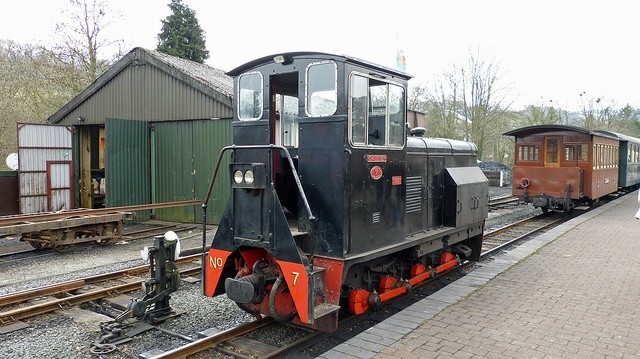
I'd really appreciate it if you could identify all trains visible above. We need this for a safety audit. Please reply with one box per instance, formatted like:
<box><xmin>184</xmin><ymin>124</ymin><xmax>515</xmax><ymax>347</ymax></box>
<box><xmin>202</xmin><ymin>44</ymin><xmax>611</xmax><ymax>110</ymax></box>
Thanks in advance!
<box><xmin>202</xmin><ymin>51</ymin><xmax>490</xmax><ymax>334</ymax></box>
<box><xmin>502</xmin><ymin>124</ymin><xmax>640</xmax><ymax>217</ymax></box>
